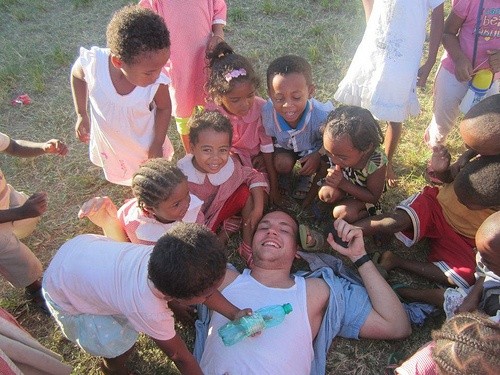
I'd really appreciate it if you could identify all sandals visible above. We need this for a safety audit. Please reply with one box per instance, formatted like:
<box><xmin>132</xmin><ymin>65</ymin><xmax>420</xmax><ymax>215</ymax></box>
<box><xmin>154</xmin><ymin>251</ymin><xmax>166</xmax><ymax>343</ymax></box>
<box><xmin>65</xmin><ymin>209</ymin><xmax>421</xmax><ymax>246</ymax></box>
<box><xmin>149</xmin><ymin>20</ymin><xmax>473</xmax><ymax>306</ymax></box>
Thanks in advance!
<box><xmin>240</xmin><ymin>243</ymin><xmax>254</xmax><ymax>269</ymax></box>
<box><xmin>25</xmin><ymin>285</ymin><xmax>51</xmax><ymax>314</ymax></box>
<box><xmin>292</xmin><ymin>176</ymin><xmax>314</xmax><ymax>200</ymax></box>
<box><xmin>221</xmin><ymin>215</ymin><xmax>240</xmax><ymax>233</ymax></box>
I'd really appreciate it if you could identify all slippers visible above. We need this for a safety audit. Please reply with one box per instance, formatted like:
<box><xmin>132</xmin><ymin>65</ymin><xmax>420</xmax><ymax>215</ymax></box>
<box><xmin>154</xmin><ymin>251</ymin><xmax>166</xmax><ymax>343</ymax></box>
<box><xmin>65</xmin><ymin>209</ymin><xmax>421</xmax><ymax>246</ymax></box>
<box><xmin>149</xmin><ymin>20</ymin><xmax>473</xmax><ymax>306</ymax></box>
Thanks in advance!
<box><xmin>298</xmin><ymin>223</ymin><xmax>324</xmax><ymax>252</ymax></box>
<box><xmin>391</xmin><ymin>281</ymin><xmax>410</xmax><ymax>302</ymax></box>
<box><xmin>373</xmin><ymin>254</ymin><xmax>389</xmax><ymax>280</ymax></box>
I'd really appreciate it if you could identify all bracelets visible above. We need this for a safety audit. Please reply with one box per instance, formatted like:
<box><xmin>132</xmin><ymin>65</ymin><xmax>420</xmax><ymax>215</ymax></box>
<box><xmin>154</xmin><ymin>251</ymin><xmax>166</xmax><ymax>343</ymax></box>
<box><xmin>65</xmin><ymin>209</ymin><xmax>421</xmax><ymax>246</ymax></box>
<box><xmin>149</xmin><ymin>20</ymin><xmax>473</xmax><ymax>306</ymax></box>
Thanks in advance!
<box><xmin>212</xmin><ymin>35</ymin><xmax>225</xmax><ymax>41</ymax></box>
<box><xmin>353</xmin><ymin>255</ymin><xmax>372</xmax><ymax>269</ymax></box>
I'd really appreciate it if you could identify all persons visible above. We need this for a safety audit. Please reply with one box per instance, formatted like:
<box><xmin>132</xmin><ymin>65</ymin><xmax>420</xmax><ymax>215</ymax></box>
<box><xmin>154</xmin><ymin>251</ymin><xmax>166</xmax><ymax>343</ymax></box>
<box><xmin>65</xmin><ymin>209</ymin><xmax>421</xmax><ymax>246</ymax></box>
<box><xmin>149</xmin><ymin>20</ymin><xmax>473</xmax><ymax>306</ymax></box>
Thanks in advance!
<box><xmin>133</xmin><ymin>0</ymin><xmax>228</xmax><ymax>161</ymax></box>
<box><xmin>332</xmin><ymin>0</ymin><xmax>449</xmax><ymax>190</ymax></box>
<box><xmin>68</xmin><ymin>4</ymin><xmax>179</xmax><ymax>201</ymax></box>
<box><xmin>421</xmin><ymin>0</ymin><xmax>500</xmax><ymax>184</ymax></box>
<box><xmin>315</xmin><ymin>104</ymin><xmax>389</xmax><ymax>226</ymax></box>
<box><xmin>40</xmin><ymin>43</ymin><xmax>500</xmax><ymax>374</ymax></box>
<box><xmin>0</xmin><ymin>133</ymin><xmax>74</xmax><ymax>316</ymax></box>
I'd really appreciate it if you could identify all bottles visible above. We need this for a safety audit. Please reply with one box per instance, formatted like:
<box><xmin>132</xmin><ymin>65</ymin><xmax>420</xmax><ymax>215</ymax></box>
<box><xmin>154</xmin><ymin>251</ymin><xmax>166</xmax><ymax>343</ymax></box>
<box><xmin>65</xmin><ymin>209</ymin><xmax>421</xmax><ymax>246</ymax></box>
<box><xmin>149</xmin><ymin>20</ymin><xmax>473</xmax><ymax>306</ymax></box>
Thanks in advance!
<box><xmin>458</xmin><ymin>69</ymin><xmax>493</xmax><ymax>114</ymax></box>
<box><xmin>218</xmin><ymin>303</ymin><xmax>292</xmax><ymax>347</ymax></box>
